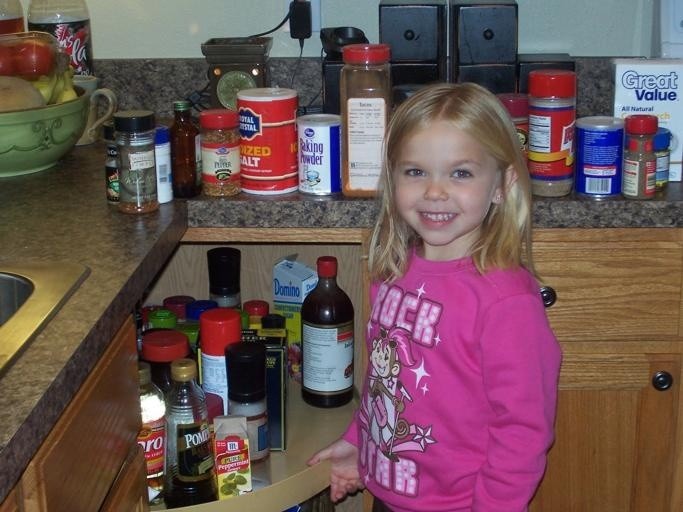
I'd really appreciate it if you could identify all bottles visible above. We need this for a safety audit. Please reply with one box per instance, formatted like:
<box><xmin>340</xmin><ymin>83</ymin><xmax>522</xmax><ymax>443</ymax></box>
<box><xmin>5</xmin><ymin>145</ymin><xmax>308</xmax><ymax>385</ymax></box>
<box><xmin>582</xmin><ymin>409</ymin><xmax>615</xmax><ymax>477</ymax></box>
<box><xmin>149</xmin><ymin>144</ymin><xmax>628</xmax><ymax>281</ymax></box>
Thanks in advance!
<box><xmin>226</xmin><ymin>341</ymin><xmax>271</xmax><ymax>465</ymax></box>
<box><xmin>172</xmin><ymin>99</ymin><xmax>198</xmax><ymax>198</ymax></box>
<box><xmin>236</xmin><ymin>89</ymin><xmax>301</xmax><ymax>196</ymax></box>
<box><xmin>243</xmin><ymin>299</ymin><xmax>267</xmax><ymax>330</ymax></box>
<box><xmin>141</xmin><ymin>329</ymin><xmax>197</xmax><ymax>397</ymax></box>
<box><xmin>148</xmin><ymin>309</ymin><xmax>177</xmax><ymax>330</ymax></box>
<box><xmin>155</xmin><ymin>126</ymin><xmax>174</xmax><ymax>205</ymax></box>
<box><xmin>28</xmin><ymin>1</ymin><xmax>92</xmax><ymax>79</ymax></box>
<box><xmin>297</xmin><ymin>114</ymin><xmax>339</xmax><ymax>196</ymax></box>
<box><xmin>529</xmin><ymin>69</ymin><xmax>577</xmax><ymax>197</ymax></box>
<box><xmin>576</xmin><ymin>115</ymin><xmax>624</xmax><ymax>198</ymax></box>
<box><xmin>340</xmin><ymin>45</ymin><xmax>393</xmax><ymax>197</ymax></box>
<box><xmin>0</xmin><ymin>1</ymin><xmax>24</xmax><ymax>33</ymax></box>
<box><xmin>105</xmin><ymin>121</ymin><xmax>121</xmax><ymax>201</ymax></box>
<box><xmin>201</xmin><ymin>310</ymin><xmax>238</xmax><ymax>416</ymax></box>
<box><xmin>623</xmin><ymin>116</ymin><xmax>657</xmax><ymax>198</ymax></box>
<box><xmin>200</xmin><ymin>111</ymin><xmax>240</xmax><ymax>197</ymax></box>
<box><xmin>207</xmin><ymin>246</ymin><xmax>241</xmax><ymax>306</ymax></box>
<box><xmin>498</xmin><ymin>92</ymin><xmax>530</xmax><ymax>158</ymax></box>
<box><xmin>186</xmin><ymin>299</ymin><xmax>216</xmax><ymax>325</ymax></box>
<box><xmin>179</xmin><ymin>323</ymin><xmax>198</xmax><ymax>349</ymax></box>
<box><xmin>262</xmin><ymin>315</ymin><xmax>286</xmax><ymax>352</ymax></box>
<box><xmin>652</xmin><ymin>126</ymin><xmax>669</xmax><ymax>192</ymax></box>
<box><xmin>164</xmin><ymin>294</ymin><xmax>194</xmax><ymax>320</ymax></box>
<box><xmin>203</xmin><ymin>394</ymin><xmax>223</xmax><ymax>434</ymax></box>
<box><xmin>166</xmin><ymin>358</ymin><xmax>218</xmax><ymax>508</ymax></box>
<box><xmin>301</xmin><ymin>255</ymin><xmax>354</xmax><ymax>407</ymax></box>
<box><xmin>116</xmin><ymin>112</ymin><xmax>157</xmax><ymax>216</ymax></box>
<box><xmin>137</xmin><ymin>363</ymin><xmax>168</xmax><ymax>503</ymax></box>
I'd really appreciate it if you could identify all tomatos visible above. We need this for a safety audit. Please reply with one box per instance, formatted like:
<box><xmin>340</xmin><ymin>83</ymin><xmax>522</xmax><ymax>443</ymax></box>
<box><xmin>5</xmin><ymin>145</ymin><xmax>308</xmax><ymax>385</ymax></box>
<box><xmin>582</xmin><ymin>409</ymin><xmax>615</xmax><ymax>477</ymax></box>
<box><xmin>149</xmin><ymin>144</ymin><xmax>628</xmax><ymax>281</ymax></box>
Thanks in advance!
<box><xmin>0</xmin><ymin>45</ymin><xmax>14</xmax><ymax>75</ymax></box>
<box><xmin>12</xmin><ymin>38</ymin><xmax>54</xmax><ymax>80</ymax></box>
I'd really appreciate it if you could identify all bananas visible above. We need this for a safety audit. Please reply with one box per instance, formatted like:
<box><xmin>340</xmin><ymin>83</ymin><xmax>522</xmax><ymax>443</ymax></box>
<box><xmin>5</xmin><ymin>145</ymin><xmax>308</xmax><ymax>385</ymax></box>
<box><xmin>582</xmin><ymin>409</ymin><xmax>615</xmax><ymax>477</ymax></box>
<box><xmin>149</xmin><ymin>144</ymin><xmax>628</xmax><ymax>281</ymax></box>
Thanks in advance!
<box><xmin>30</xmin><ymin>55</ymin><xmax>78</xmax><ymax>104</ymax></box>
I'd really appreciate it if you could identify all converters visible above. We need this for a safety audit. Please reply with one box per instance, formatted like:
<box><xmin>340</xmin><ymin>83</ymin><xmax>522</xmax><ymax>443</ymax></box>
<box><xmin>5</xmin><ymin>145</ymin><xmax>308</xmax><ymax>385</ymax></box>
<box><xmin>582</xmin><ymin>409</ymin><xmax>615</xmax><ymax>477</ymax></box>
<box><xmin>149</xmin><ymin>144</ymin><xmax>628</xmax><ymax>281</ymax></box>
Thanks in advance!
<box><xmin>290</xmin><ymin>2</ymin><xmax>312</xmax><ymax>38</ymax></box>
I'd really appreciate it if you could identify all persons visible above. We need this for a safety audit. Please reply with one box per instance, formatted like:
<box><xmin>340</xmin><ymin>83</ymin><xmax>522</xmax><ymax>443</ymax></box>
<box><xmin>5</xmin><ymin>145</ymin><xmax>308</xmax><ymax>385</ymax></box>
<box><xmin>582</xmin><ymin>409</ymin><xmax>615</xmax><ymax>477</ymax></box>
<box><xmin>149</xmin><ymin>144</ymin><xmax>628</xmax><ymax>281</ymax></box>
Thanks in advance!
<box><xmin>304</xmin><ymin>80</ymin><xmax>566</xmax><ymax>512</ymax></box>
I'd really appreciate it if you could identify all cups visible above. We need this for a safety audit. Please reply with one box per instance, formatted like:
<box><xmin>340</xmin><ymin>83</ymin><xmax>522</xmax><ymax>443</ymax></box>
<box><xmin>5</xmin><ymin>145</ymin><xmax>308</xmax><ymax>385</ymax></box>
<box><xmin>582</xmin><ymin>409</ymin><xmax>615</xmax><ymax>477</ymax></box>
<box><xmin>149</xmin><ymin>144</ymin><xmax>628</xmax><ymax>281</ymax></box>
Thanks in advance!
<box><xmin>68</xmin><ymin>72</ymin><xmax>120</xmax><ymax>147</ymax></box>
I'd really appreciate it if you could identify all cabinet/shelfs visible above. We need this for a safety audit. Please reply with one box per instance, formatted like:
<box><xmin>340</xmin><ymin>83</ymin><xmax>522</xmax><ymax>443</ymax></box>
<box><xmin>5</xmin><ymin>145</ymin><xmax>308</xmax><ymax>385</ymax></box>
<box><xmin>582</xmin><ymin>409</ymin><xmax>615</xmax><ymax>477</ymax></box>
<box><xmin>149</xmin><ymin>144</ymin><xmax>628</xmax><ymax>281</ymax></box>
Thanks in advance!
<box><xmin>0</xmin><ymin>229</ymin><xmax>376</xmax><ymax>512</ymax></box>
<box><xmin>521</xmin><ymin>229</ymin><xmax>682</xmax><ymax>512</ymax></box>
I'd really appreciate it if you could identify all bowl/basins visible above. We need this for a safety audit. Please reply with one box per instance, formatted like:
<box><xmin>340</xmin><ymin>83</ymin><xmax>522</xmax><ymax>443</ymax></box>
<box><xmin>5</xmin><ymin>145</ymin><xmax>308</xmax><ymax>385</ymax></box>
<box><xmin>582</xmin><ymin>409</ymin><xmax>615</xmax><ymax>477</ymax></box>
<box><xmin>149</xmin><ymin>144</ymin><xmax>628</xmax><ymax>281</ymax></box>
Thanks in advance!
<box><xmin>0</xmin><ymin>91</ymin><xmax>91</xmax><ymax>178</ymax></box>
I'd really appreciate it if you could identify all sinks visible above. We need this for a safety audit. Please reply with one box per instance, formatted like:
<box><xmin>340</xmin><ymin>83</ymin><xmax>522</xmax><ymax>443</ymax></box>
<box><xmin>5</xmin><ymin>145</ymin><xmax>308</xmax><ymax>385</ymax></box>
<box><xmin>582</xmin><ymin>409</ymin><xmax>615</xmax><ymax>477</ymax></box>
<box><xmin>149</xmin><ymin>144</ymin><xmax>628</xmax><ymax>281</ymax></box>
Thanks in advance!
<box><xmin>0</xmin><ymin>256</ymin><xmax>92</xmax><ymax>378</ymax></box>
